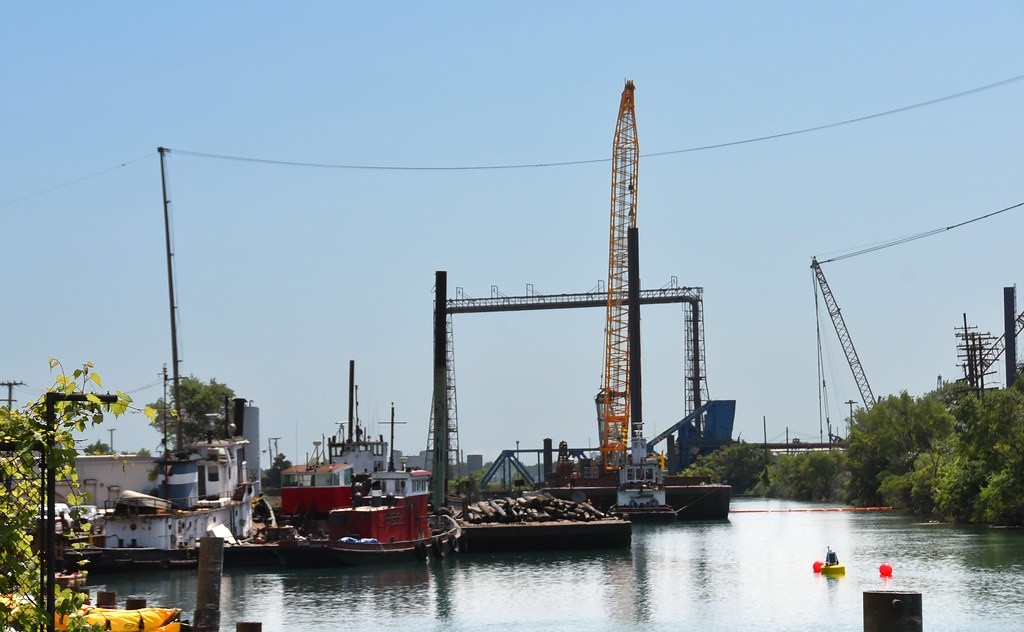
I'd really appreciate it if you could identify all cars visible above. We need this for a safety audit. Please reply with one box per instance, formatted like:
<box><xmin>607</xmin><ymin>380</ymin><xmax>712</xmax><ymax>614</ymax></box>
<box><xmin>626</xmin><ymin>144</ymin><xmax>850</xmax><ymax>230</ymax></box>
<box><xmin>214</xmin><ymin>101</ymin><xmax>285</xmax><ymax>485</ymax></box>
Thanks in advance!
<box><xmin>33</xmin><ymin>502</ymin><xmax>117</xmax><ymax>532</ymax></box>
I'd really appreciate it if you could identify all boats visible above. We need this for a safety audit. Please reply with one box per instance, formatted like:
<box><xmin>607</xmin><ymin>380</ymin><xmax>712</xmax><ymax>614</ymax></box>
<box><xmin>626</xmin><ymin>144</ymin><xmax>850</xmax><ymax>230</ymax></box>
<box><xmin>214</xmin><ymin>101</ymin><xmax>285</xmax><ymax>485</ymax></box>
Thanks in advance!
<box><xmin>67</xmin><ymin>412</ymin><xmax>283</xmax><ymax>575</ymax></box>
<box><xmin>322</xmin><ymin>403</ymin><xmax>460</xmax><ymax>565</ymax></box>
<box><xmin>607</xmin><ymin>430</ymin><xmax>681</xmax><ymax>521</ymax></box>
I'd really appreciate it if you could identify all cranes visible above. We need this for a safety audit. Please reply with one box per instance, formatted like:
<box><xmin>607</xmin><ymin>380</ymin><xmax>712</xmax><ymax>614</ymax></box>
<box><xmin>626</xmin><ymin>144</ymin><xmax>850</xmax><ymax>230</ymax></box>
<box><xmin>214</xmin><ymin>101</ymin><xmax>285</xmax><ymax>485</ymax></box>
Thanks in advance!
<box><xmin>811</xmin><ymin>255</ymin><xmax>876</xmax><ymax>412</ymax></box>
<box><xmin>586</xmin><ymin>77</ymin><xmax>669</xmax><ymax>477</ymax></box>
